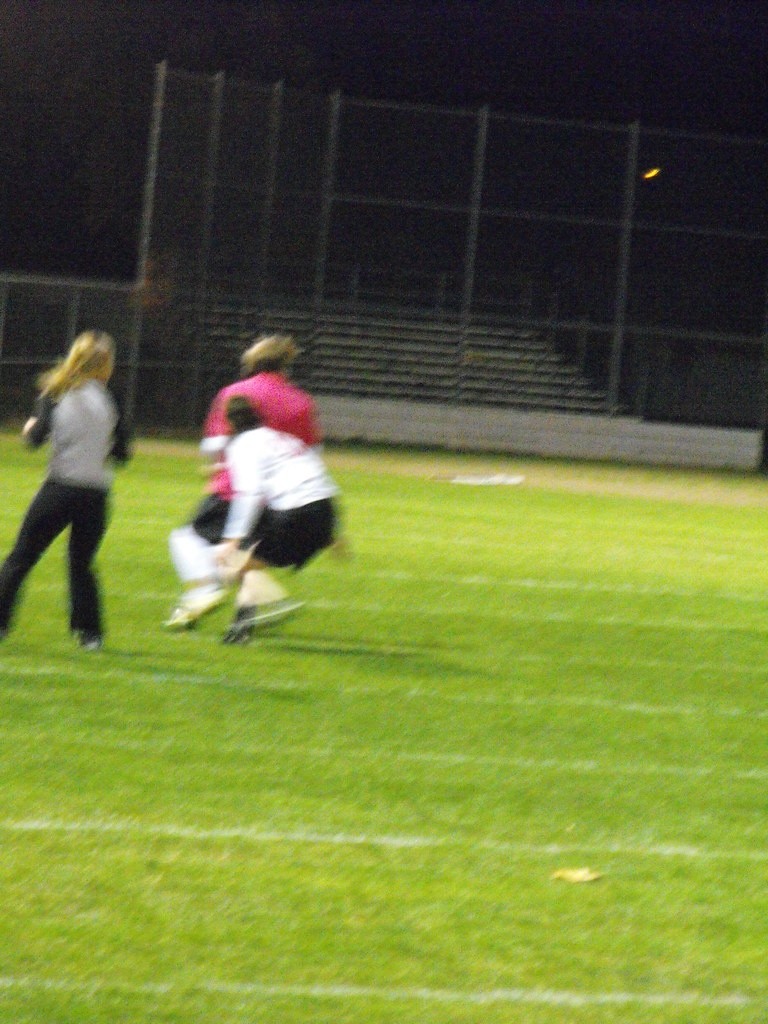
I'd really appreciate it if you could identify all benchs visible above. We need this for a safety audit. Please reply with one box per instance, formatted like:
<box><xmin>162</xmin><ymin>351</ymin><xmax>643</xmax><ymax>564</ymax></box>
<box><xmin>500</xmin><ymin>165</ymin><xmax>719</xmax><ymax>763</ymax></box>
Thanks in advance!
<box><xmin>188</xmin><ymin>302</ymin><xmax>631</xmax><ymax>413</ymax></box>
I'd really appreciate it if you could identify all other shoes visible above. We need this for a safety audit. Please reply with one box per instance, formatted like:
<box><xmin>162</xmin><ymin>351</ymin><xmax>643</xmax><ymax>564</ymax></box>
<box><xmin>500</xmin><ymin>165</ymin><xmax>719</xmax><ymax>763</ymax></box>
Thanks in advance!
<box><xmin>79</xmin><ymin>631</ymin><xmax>100</xmax><ymax>653</ymax></box>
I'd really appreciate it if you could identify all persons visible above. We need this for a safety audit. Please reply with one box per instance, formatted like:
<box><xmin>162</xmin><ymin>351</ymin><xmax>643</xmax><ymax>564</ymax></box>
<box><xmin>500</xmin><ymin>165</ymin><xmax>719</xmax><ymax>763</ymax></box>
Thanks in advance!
<box><xmin>166</xmin><ymin>334</ymin><xmax>338</xmax><ymax>642</ymax></box>
<box><xmin>1</xmin><ymin>331</ymin><xmax>131</xmax><ymax>649</ymax></box>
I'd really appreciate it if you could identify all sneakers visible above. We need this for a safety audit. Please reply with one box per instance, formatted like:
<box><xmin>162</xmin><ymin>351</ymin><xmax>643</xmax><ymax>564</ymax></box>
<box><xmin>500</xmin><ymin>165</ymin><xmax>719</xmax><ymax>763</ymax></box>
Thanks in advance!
<box><xmin>164</xmin><ymin>584</ymin><xmax>226</xmax><ymax>630</ymax></box>
